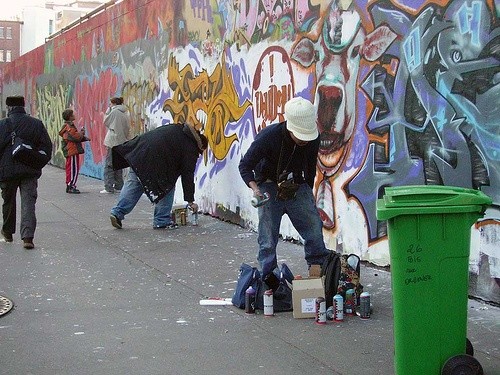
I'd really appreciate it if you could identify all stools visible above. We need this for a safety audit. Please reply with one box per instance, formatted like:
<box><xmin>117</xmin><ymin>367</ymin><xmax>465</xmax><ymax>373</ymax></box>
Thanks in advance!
<box><xmin>172</xmin><ymin>202</ymin><xmax>189</xmax><ymax>223</ymax></box>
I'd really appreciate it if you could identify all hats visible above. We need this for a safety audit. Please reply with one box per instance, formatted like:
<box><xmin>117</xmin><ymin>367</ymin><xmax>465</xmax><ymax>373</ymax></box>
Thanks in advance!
<box><xmin>62</xmin><ymin>108</ymin><xmax>73</xmax><ymax>120</ymax></box>
<box><xmin>283</xmin><ymin>97</ymin><xmax>319</xmax><ymax>141</ymax></box>
<box><xmin>6</xmin><ymin>95</ymin><xmax>25</xmax><ymax>107</ymax></box>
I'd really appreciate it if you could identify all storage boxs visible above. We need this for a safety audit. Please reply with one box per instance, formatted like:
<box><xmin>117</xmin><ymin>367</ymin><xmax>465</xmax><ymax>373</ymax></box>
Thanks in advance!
<box><xmin>292</xmin><ymin>267</ymin><xmax>325</xmax><ymax>319</ymax></box>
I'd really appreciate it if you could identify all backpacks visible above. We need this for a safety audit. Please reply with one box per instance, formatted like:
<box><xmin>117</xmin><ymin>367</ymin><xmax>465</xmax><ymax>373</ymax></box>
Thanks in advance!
<box><xmin>319</xmin><ymin>250</ymin><xmax>364</xmax><ymax>311</ymax></box>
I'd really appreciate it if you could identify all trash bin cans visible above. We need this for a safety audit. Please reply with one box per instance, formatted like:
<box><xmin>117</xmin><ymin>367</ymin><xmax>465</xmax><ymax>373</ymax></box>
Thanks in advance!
<box><xmin>377</xmin><ymin>184</ymin><xmax>493</xmax><ymax>375</ymax></box>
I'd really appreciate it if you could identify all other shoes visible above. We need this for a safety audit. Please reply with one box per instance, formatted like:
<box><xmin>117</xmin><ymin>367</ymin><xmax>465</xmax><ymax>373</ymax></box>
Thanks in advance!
<box><xmin>100</xmin><ymin>188</ymin><xmax>121</xmax><ymax>194</ymax></box>
<box><xmin>66</xmin><ymin>187</ymin><xmax>80</xmax><ymax>194</ymax></box>
<box><xmin>24</xmin><ymin>238</ymin><xmax>34</xmax><ymax>248</ymax></box>
<box><xmin>110</xmin><ymin>214</ymin><xmax>122</xmax><ymax>229</ymax></box>
<box><xmin>1</xmin><ymin>230</ymin><xmax>13</xmax><ymax>242</ymax></box>
<box><xmin>153</xmin><ymin>222</ymin><xmax>178</xmax><ymax>230</ymax></box>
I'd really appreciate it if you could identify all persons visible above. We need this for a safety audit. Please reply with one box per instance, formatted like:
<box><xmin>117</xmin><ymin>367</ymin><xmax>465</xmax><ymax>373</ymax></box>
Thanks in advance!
<box><xmin>239</xmin><ymin>96</ymin><xmax>328</xmax><ymax>276</ymax></box>
<box><xmin>100</xmin><ymin>97</ymin><xmax>130</xmax><ymax>194</ymax></box>
<box><xmin>0</xmin><ymin>95</ymin><xmax>52</xmax><ymax>247</ymax></box>
<box><xmin>110</xmin><ymin>123</ymin><xmax>208</xmax><ymax>230</ymax></box>
<box><xmin>58</xmin><ymin>109</ymin><xmax>91</xmax><ymax>193</ymax></box>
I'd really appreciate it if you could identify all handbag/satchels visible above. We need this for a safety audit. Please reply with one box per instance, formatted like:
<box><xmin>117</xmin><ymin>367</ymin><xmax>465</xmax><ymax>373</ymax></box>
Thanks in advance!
<box><xmin>275</xmin><ymin>170</ymin><xmax>299</xmax><ymax>201</ymax></box>
<box><xmin>255</xmin><ymin>263</ymin><xmax>294</xmax><ymax>312</ymax></box>
<box><xmin>6</xmin><ymin>117</ymin><xmax>50</xmax><ymax>169</ymax></box>
<box><xmin>232</xmin><ymin>262</ymin><xmax>261</xmax><ymax>309</ymax></box>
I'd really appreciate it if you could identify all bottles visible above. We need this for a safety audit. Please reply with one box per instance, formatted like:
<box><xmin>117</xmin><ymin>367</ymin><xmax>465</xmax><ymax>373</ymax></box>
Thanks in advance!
<box><xmin>252</xmin><ymin>191</ymin><xmax>271</xmax><ymax>208</ymax></box>
<box><xmin>345</xmin><ymin>289</ymin><xmax>356</xmax><ymax>316</ymax></box>
<box><xmin>315</xmin><ymin>296</ymin><xmax>327</xmax><ymax>324</ymax></box>
<box><xmin>326</xmin><ymin>306</ymin><xmax>333</xmax><ymax>320</ymax></box>
<box><xmin>191</xmin><ymin>212</ymin><xmax>198</xmax><ymax>226</ymax></box>
<box><xmin>244</xmin><ymin>286</ymin><xmax>256</xmax><ymax>315</ymax></box>
<box><xmin>263</xmin><ymin>289</ymin><xmax>274</xmax><ymax>317</ymax></box>
<box><xmin>360</xmin><ymin>292</ymin><xmax>370</xmax><ymax>318</ymax></box>
<box><xmin>356</xmin><ymin>303</ymin><xmax>375</xmax><ymax>316</ymax></box>
<box><xmin>181</xmin><ymin>212</ymin><xmax>187</xmax><ymax>225</ymax></box>
<box><xmin>333</xmin><ymin>292</ymin><xmax>344</xmax><ymax>322</ymax></box>
<box><xmin>81</xmin><ymin>126</ymin><xmax>85</xmax><ymax>135</ymax></box>
